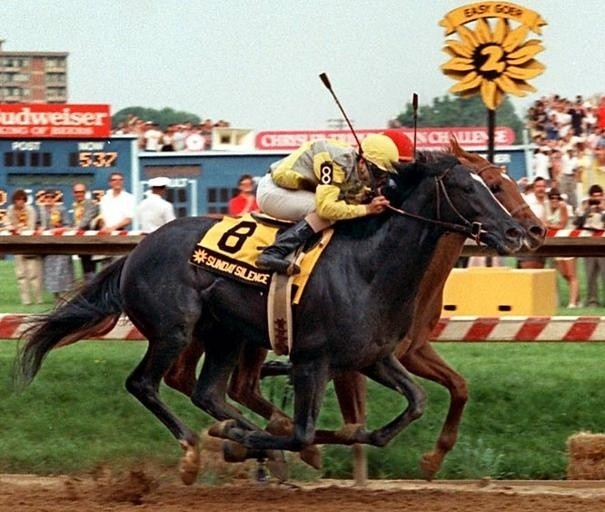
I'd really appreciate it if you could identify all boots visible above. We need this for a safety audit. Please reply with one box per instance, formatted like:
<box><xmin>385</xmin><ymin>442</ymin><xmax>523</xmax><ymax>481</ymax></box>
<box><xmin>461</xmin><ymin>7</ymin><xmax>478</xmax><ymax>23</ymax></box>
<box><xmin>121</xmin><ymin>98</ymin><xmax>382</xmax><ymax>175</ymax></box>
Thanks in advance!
<box><xmin>253</xmin><ymin>219</ymin><xmax>317</xmax><ymax>277</ymax></box>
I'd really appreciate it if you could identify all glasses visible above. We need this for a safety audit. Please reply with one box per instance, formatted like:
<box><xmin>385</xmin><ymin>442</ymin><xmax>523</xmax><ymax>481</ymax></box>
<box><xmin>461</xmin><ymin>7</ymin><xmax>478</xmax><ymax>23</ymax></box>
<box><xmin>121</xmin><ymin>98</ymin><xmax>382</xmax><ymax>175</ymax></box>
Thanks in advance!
<box><xmin>551</xmin><ymin>197</ymin><xmax>562</xmax><ymax>201</ymax></box>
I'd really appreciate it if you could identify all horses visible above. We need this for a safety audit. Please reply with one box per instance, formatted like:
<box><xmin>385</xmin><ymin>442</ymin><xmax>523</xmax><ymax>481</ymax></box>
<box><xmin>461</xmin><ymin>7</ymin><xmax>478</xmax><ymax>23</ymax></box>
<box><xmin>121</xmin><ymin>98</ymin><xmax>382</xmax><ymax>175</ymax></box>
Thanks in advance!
<box><xmin>15</xmin><ymin>149</ymin><xmax>528</xmax><ymax>486</ymax></box>
<box><xmin>163</xmin><ymin>129</ymin><xmax>547</xmax><ymax>484</ymax></box>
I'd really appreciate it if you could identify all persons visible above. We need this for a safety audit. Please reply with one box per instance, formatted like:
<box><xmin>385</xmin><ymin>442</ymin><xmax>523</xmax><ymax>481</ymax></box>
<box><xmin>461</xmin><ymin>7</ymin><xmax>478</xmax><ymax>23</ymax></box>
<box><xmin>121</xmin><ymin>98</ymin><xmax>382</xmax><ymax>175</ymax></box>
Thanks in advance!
<box><xmin>1</xmin><ymin>188</ymin><xmax>44</xmax><ymax>305</ymax></box>
<box><xmin>255</xmin><ymin>134</ymin><xmax>401</xmax><ymax>275</ymax></box>
<box><xmin>453</xmin><ymin>176</ymin><xmax>605</xmax><ymax>309</ymax></box>
<box><xmin>35</xmin><ymin>190</ymin><xmax>76</xmax><ymax>302</ymax></box>
<box><xmin>71</xmin><ymin>183</ymin><xmax>102</xmax><ymax>281</ymax></box>
<box><xmin>525</xmin><ymin>93</ymin><xmax>605</xmax><ymax>218</ymax></box>
<box><xmin>99</xmin><ymin>173</ymin><xmax>135</xmax><ymax>268</ymax></box>
<box><xmin>109</xmin><ymin>114</ymin><xmax>231</xmax><ymax>152</ymax></box>
<box><xmin>135</xmin><ymin>176</ymin><xmax>176</xmax><ymax>234</ymax></box>
<box><xmin>228</xmin><ymin>173</ymin><xmax>259</xmax><ymax>216</ymax></box>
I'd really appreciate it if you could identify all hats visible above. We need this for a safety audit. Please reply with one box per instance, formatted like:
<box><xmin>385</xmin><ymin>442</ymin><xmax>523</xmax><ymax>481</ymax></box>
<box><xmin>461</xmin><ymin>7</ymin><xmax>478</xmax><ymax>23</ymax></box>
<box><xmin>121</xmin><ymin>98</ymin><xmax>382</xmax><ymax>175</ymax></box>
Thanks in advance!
<box><xmin>549</xmin><ymin>187</ymin><xmax>562</xmax><ymax>196</ymax></box>
<box><xmin>148</xmin><ymin>176</ymin><xmax>170</xmax><ymax>188</ymax></box>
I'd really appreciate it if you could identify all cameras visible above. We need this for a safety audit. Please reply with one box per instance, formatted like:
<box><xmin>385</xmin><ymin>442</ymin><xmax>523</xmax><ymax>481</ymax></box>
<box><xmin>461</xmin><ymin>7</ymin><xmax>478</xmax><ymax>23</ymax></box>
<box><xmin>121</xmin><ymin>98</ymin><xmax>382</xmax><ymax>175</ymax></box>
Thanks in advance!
<box><xmin>588</xmin><ymin>199</ymin><xmax>601</xmax><ymax>206</ymax></box>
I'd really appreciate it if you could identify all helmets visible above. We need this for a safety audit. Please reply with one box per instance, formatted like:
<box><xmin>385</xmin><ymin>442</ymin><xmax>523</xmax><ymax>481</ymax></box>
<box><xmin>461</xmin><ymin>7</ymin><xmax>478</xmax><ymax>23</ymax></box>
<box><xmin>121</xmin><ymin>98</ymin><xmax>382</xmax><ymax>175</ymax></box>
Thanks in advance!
<box><xmin>380</xmin><ymin>127</ymin><xmax>419</xmax><ymax>161</ymax></box>
<box><xmin>354</xmin><ymin>134</ymin><xmax>400</xmax><ymax>175</ymax></box>
